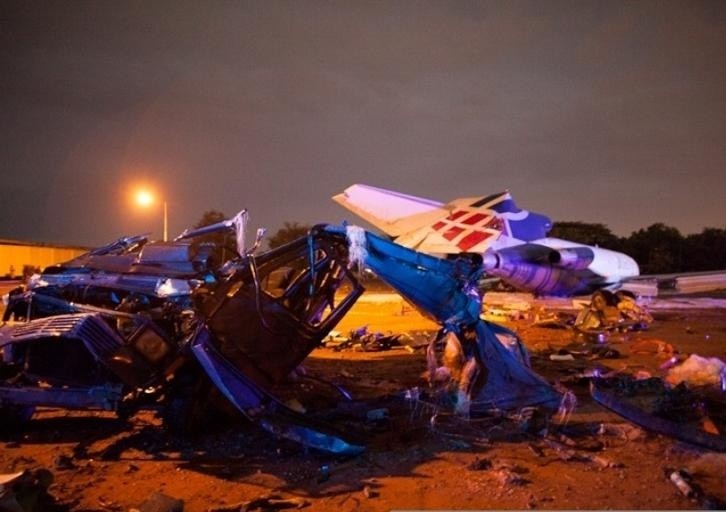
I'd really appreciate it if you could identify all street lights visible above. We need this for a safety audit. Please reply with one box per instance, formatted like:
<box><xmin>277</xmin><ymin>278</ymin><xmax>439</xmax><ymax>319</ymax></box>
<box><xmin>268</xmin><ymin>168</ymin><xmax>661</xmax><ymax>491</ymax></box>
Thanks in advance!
<box><xmin>135</xmin><ymin>188</ymin><xmax>168</xmax><ymax>242</ymax></box>
